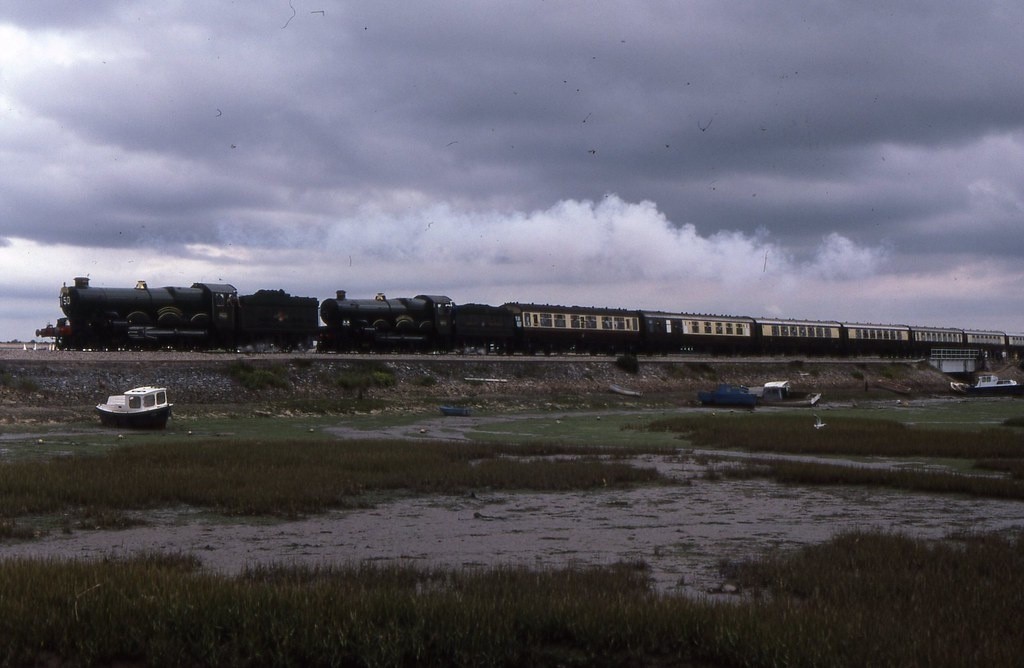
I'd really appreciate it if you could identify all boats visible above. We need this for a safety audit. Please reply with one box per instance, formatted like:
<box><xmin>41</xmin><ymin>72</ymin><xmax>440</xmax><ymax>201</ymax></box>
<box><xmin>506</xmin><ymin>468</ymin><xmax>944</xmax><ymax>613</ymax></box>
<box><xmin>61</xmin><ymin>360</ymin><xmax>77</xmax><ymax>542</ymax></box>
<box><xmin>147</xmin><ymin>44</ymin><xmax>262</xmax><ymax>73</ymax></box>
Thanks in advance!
<box><xmin>949</xmin><ymin>375</ymin><xmax>1024</xmax><ymax>398</ymax></box>
<box><xmin>94</xmin><ymin>386</ymin><xmax>173</xmax><ymax>431</ymax></box>
<box><xmin>696</xmin><ymin>382</ymin><xmax>758</xmax><ymax>408</ymax></box>
<box><xmin>746</xmin><ymin>381</ymin><xmax>823</xmax><ymax>408</ymax></box>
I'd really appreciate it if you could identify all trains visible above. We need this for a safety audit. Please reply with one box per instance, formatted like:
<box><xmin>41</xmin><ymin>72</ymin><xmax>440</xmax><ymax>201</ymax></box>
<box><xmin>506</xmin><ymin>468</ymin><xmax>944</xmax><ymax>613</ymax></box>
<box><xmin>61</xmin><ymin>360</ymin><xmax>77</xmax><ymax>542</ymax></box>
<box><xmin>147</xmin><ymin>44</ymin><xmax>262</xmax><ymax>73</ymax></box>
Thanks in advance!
<box><xmin>34</xmin><ymin>276</ymin><xmax>1024</xmax><ymax>357</ymax></box>
